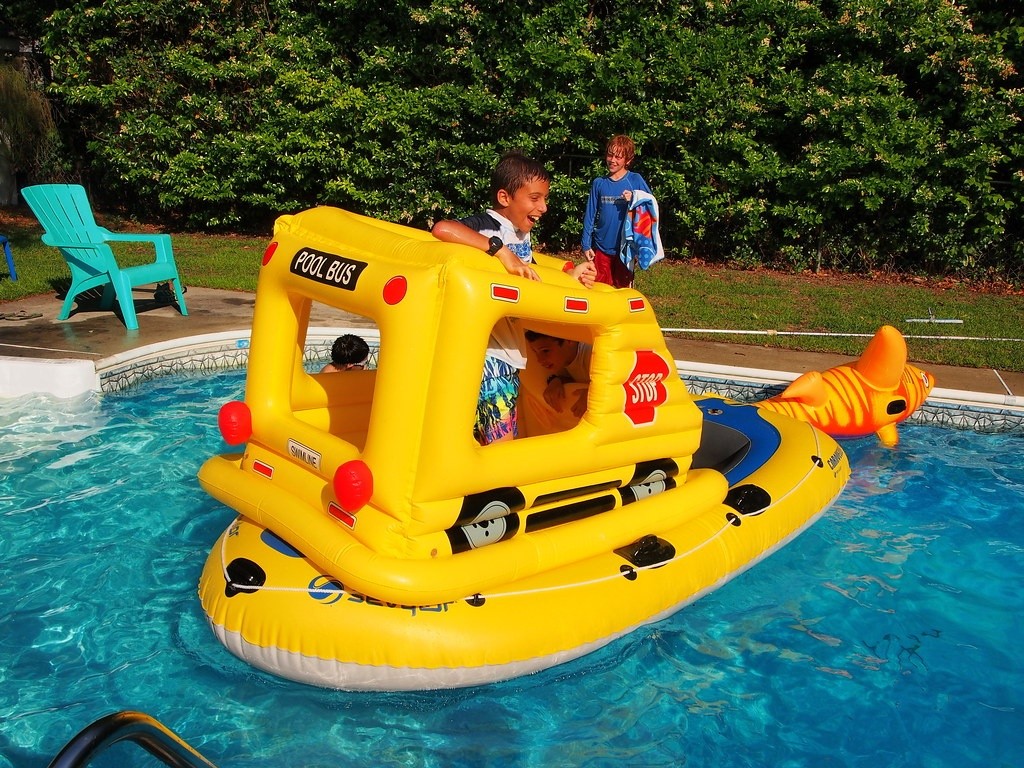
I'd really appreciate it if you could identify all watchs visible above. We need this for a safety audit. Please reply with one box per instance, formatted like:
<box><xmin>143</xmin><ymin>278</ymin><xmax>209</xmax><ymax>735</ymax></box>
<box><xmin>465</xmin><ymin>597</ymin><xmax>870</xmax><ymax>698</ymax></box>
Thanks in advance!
<box><xmin>484</xmin><ymin>234</ymin><xmax>503</xmax><ymax>255</ymax></box>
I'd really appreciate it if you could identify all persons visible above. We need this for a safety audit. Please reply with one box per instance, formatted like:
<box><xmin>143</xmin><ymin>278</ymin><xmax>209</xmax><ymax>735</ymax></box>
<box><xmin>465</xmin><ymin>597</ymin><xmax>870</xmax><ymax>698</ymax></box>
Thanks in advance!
<box><xmin>523</xmin><ymin>328</ymin><xmax>592</xmax><ymax>416</ymax></box>
<box><xmin>581</xmin><ymin>134</ymin><xmax>653</xmax><ymax>288</ymax></box>
<box><xmin>430</xmin><ymin>152</ymin><xmax>596</xmax><ymax>446</ymax></box>
<box><xmin>318</xmin><ymin>334</ymin><xmax>369</xmax><ymax>372</ymax></box>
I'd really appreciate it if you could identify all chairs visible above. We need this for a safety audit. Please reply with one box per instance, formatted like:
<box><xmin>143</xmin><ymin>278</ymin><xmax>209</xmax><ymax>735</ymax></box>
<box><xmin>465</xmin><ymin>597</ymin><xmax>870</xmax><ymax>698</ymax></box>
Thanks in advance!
<box><xmin>20</xmin><ymin>184</ymin><xmax>188</xmax><ymax>331</ymax></box>
